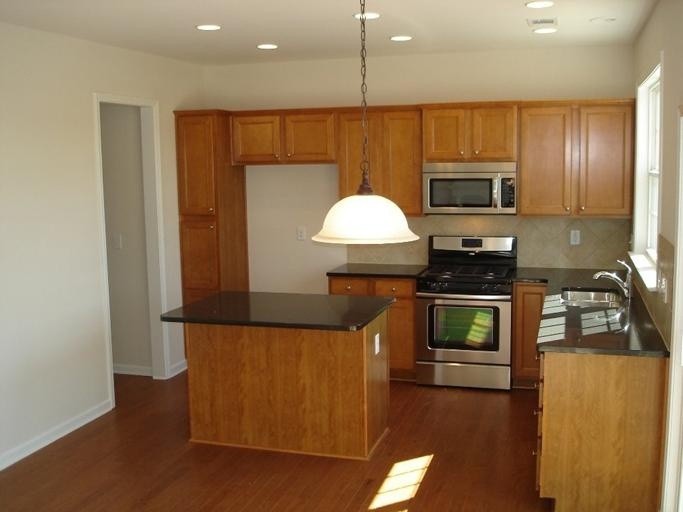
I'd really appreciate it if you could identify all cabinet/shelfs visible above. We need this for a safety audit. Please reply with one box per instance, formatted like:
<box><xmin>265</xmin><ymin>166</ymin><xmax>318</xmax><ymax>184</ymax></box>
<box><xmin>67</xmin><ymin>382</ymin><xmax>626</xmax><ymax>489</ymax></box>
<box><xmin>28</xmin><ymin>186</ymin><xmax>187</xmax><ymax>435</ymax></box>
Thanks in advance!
<box><xmin>520</xmin><ymin>106</ymin><xmax>632</xmax><ymax>215</ymax></box>
<box><xmin>176</xmin><ymin>115</ymin><xmax>219</xmax><ymax>291</ymax></box>
<box><xmin>160</xmin><ymin>290</ymin><xmax>396</xmax><ymax>461</ymax></box>
<box><xmin>330</xmin><ymin>279</ymin><xmax>416</xmax><ymax>378</ymax></box>
<box><xmin>513</xmin><ymin>283</ymin><xmax>547</xmax><ymax>380</ymax></box>
<box><xmin>532</xmin><ymin>352</ymin><xmax>669</xmax><ymax>512</ymax></box>
<box><xmin>231</xmin><ymin>113</ymin><xmax>335</xmax><ymax>161</ymax></box>
<box><xmin>422</xmin><ymin>104</ymin><xmax>517</xmax><ymax>161</ymax></box>
<box><xmin>340</xmin><ymin>111</ymin><xmax>421</xmax><ymax>214</ymax></box>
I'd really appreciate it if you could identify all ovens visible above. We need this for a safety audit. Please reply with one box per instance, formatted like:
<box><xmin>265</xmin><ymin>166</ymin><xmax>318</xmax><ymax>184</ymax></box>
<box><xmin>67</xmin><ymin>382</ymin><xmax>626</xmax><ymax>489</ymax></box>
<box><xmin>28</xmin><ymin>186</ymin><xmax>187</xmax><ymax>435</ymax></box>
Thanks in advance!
<box><xmin>412</xmin><ymin>294</ymin><xmax>511</xmax><ymax>391</ymax></box>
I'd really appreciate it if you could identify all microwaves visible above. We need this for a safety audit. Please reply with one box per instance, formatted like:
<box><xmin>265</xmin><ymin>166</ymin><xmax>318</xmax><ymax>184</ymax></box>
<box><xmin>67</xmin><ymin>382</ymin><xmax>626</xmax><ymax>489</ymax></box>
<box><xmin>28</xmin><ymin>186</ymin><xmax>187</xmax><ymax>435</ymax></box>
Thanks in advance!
<box><xmin>421</xmin><ymin>162</ymin><xmax>518</xmax><ymax>217</ymax></box>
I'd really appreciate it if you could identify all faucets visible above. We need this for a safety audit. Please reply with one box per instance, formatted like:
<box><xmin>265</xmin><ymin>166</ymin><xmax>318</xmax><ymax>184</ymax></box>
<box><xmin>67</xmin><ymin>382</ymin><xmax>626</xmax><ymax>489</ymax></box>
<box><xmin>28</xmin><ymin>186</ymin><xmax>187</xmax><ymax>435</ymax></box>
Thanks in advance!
<box><xmin>593</xmin><ymin>300</ymin><xmax>632</xmax><ymax>335</ymax></box>
<box><xmin>593</xmin><ymin>259</ymin><xmax>633</xmax><ymax>300</ymax></box>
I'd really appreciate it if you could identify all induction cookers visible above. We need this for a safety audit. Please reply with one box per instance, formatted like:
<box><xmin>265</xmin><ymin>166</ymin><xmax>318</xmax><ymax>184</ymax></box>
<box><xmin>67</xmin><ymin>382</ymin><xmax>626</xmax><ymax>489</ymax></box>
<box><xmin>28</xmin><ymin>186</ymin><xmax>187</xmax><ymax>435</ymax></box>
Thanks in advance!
<box><xmin>415</xmin><ymin>264</ymin><xmax>512</xmax><ymax>293</ymax></box>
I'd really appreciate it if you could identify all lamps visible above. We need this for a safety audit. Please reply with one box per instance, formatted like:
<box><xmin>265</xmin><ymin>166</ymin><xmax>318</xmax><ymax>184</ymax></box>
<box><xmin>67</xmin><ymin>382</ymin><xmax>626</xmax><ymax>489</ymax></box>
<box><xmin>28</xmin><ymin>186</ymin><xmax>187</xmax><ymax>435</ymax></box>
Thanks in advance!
<box><xmin>311</xmin><ymin>0</ymin><xmax>420</xmax><ymax>245</ymax></box>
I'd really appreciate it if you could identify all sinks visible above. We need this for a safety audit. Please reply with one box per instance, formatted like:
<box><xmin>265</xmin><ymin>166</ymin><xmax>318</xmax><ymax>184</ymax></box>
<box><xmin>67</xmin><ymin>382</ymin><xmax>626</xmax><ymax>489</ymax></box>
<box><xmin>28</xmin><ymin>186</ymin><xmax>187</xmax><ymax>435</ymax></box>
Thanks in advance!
<box><xmin>561</xmin><ymin>286</ymin><xmax>624</xmax><ymax>303</ymax></box>
